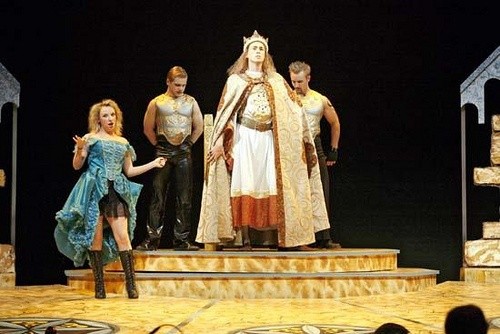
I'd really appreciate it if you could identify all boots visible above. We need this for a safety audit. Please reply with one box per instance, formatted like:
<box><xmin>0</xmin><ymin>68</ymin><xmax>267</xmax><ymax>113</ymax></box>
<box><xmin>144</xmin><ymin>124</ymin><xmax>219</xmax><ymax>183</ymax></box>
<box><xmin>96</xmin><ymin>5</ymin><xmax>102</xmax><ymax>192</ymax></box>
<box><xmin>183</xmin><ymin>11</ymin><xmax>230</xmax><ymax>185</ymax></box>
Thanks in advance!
<box><xmin>87</xmin><ymin>250</ymin><xmax>106</xmax><ymax>299</ymax></box>
<box><xmin>172</xmin><ymin>229</ymin><xmax>199</xmax><ymax>251</ymax></box>
<box><xmin>145</xmin><ymin>223</ymin><xmax>163</xmax><ymax>251</ymax></box>
<box><xmin>118</xmin><ymin>250</ymin><xmax>138</xmax><ymax>298</ymax></box>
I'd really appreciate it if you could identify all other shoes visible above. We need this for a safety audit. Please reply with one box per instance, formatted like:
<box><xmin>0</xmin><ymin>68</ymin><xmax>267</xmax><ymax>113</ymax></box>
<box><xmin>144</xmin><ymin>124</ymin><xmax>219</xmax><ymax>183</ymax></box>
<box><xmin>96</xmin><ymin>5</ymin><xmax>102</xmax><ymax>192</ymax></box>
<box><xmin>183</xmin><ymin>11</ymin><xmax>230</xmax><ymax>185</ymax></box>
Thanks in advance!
<box><xmin>317</xmin><ymin>239</ymin><xmax>340</xmax><ymax>249</ymax></box>
<box><xmin>263</xmin><ymin>240</ymin><xmax>276</xmax><ymax>246</ymax></box>
<box><xmin>239</xmin><ymin>243</ymin><xmax>252</xmax><ymax>251</ymax></box>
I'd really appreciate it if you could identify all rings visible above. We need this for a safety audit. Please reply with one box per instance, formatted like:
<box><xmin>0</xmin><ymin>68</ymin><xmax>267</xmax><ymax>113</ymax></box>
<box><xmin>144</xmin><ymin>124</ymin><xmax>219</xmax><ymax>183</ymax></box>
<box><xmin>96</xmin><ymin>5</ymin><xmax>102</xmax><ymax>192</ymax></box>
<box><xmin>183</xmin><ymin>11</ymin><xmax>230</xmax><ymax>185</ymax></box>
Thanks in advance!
<box><xmin>209</xmin><ymin>151</ymin><xmax>212</xmax><ymax>154</ymax></box>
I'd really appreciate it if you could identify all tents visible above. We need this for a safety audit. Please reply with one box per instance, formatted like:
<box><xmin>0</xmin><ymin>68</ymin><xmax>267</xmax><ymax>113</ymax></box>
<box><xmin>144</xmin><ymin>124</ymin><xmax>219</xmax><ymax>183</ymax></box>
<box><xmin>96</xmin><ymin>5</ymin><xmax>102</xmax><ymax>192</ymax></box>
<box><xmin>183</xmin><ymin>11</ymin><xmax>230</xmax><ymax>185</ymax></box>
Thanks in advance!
<box><xmin>459</xmin><ymin>44</ymin><xmax>500</xmax><ymax>268</ymax></box>
<box><xmin>0</xmin><ymin>63</ymin><xmax>20</xmax><ymax>250</ymax></box>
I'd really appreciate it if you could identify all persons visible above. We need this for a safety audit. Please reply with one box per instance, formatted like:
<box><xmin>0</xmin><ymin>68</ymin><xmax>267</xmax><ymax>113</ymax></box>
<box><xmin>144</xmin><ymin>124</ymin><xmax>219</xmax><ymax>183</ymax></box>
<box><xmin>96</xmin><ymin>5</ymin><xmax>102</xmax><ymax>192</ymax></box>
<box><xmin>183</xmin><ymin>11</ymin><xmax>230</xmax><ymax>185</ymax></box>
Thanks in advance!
<box><xmin>195</xmin><ymin>30</ymin><xmax>331</xmax><ymax>251</ymax></box>
<box><xmin>136</xmin><ymin>65</ymin><xmax>203</xmax><ymax>251</ymax></box>
<box><xmin>53</xmin><ymin>99</ymin><xmax>168</xmax><ymax>299</ymax></box>
<box><xmin>288</xmin><ymin>61</ymin><xmax>341</xmax><ymax>250</ymax></box>
<box><xmin>374</xmin><ymin>322</ymin><xmax>410</xmax><ymax>334</ymax></box>
<box><xmin>444</xmin><ymin>304</ymin><xmax>489</xmax><ymax>334</ymax></box>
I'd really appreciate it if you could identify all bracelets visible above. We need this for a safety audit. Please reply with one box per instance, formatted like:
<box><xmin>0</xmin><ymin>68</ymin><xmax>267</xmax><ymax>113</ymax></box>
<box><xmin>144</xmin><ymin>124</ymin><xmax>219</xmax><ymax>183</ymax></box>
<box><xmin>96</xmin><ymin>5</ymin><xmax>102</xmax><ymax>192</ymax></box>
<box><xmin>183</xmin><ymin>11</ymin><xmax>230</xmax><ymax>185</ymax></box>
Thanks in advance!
<box><xmin>78</xmin><ymin>145</ymin><xmax>83</xmax><ymax>150</ymax></box>
